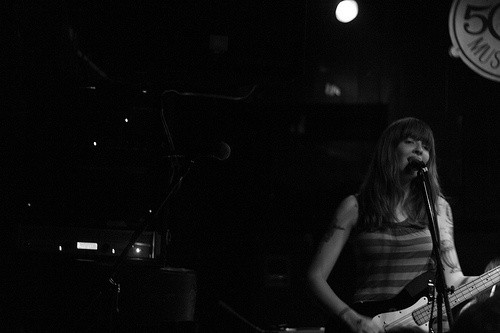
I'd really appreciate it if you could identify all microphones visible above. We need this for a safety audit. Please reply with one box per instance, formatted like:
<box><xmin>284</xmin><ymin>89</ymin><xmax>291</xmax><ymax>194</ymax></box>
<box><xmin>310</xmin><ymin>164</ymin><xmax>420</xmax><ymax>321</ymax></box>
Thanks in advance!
<box><xmin>168</xmin><ymin>142</ymin><xmax>231</xmax><ymax>160</ymax></box>
<box><xmin>409</xmin><ymin>159</ymin><xmax>427</xmax><ymax>175</ymax></box>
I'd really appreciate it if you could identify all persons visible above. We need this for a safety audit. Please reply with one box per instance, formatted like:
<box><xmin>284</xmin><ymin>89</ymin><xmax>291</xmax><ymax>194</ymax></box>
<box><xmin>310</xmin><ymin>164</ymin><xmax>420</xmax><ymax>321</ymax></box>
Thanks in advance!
<box><xmin>308</xmin><ymin>117</ymin><xmax>496</xmax><ymax>333</ymax></box>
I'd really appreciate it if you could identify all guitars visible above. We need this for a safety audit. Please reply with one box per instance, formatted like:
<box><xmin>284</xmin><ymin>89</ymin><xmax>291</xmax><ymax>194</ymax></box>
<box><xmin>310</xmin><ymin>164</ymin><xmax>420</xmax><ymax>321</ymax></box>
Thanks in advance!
<box><xmin>324</xmin><ymin>272</ymin><xmax>500</xmax><ymax>333</ymax></box>
